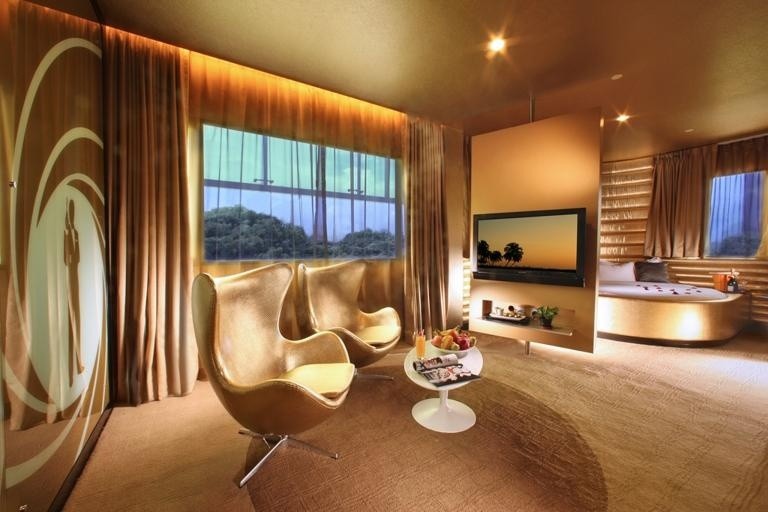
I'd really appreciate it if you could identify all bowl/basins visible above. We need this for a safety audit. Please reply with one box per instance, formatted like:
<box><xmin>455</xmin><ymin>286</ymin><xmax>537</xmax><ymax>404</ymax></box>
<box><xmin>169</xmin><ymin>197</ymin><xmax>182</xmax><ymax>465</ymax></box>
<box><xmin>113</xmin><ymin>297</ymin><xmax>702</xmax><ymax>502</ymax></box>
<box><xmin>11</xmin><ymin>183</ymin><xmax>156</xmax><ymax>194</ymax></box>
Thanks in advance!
<box><xmin>430</xmin><ymin>342</ymin><xmax>475</xmax><ymax>358</ymax></box>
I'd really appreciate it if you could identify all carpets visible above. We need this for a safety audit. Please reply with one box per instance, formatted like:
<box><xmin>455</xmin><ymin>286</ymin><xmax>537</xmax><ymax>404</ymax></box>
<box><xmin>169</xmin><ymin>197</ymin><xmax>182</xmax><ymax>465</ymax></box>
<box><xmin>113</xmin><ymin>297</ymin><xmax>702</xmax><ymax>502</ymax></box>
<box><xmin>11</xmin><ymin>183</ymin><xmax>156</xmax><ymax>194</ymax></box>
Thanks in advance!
<box><xmin>62</xmin><ymin>365</ymin><xmax>608</xmax><ymax>512</ymax></box>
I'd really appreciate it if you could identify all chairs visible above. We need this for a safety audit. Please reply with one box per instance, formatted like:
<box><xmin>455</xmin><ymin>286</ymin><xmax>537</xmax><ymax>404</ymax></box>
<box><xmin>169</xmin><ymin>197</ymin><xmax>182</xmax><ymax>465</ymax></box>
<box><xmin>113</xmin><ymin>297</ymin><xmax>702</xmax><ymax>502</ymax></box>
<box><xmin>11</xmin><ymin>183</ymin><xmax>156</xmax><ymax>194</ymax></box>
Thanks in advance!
<box><xmin>191</xmin><ymin>263</ymin><xmax>358</xmax><ymax>488</ymax></box>
<box><xmin>295</xmin><ymin>259</ymin><xmax>402</xmax><ymax>380</ymax></box>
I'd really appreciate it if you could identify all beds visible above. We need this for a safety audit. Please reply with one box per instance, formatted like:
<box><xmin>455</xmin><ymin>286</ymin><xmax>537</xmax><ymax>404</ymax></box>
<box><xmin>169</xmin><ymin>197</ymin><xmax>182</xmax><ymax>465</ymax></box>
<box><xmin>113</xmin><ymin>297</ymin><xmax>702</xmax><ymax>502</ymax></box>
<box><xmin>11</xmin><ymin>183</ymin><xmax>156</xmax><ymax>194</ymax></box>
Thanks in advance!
<box><xmin>596</xmin><ymin>280</ymin><xmax>752</xmax><ymax>349</ymax></box>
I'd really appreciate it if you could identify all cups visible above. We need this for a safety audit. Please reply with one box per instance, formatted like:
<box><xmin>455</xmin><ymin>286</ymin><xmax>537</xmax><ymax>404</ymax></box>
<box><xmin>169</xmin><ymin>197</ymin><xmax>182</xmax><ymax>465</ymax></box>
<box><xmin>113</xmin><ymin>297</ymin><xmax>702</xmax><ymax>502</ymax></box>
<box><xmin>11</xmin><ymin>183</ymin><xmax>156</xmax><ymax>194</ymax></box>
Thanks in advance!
<box><xmin>496</xmin><ymin>309</ymin><xmax>503</xmax><ymax>316</ymax></box>
<box><xmin>416</xmin><ymin>335</ymin><xmax>426</xmax><ymax>360</ymax></box>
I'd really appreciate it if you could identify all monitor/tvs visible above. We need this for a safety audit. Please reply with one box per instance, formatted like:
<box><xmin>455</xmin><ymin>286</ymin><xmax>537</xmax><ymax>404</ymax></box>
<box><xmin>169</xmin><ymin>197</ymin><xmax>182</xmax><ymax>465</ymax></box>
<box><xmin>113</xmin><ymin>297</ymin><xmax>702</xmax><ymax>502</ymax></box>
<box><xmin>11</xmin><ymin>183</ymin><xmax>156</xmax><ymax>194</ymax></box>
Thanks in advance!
<box><xmin>473</xmin><ymin>207</ymin><xmax>586</xmax><ymax>288</ymax></box>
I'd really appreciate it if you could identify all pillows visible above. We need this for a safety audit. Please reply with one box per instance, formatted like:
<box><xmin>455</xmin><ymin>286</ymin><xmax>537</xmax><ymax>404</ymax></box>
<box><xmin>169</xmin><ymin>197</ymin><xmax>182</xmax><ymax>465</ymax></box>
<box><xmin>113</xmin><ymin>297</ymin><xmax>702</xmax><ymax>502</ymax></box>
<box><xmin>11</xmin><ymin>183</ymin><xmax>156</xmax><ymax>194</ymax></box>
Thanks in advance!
<box><xmin>598</xmin><ymin>257</ymin><xmax>671</xmax><ymax>283</ymax></box>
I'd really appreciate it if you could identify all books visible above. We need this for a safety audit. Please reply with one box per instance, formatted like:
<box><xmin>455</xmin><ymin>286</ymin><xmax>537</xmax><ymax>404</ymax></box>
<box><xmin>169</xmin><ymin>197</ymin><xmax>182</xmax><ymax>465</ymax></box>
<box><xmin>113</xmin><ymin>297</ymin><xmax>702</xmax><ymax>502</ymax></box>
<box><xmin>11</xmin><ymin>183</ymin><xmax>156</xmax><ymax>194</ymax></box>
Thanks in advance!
<box><xmin>412</xmin><ymin>353</ymin><xmax>481</xmax><ymax>388</ymax></box>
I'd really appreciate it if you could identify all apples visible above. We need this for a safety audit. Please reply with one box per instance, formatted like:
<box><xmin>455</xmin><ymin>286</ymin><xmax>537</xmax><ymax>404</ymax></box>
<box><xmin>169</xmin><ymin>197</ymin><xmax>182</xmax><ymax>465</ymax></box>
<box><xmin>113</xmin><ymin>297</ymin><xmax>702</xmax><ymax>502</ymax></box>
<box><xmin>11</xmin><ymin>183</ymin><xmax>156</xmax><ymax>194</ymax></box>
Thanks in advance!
<box><xmin>456</xmin><ymin>332</ymin><xmax>470</xmax><ymax>350</ymax></box>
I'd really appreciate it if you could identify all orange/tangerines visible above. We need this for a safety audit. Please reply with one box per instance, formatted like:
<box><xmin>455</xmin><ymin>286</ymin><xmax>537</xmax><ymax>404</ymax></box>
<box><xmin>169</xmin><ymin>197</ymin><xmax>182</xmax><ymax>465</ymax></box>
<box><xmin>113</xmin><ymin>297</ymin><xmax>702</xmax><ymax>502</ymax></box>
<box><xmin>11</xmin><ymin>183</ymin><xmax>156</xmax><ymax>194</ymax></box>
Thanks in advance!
<box><xmin>431</xmin><ymin>336</ymin><xmax>442</xmax><ymax>347</ymax></box>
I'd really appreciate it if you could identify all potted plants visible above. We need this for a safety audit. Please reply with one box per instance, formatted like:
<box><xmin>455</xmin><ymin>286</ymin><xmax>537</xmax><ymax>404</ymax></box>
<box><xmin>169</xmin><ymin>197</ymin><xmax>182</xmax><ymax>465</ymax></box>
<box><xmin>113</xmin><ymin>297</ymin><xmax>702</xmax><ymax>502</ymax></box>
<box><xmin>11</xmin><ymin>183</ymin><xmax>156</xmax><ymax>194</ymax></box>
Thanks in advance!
<box><xmin>530</xmin><ymin>305</ymin><xmax>561</xmax><ymax>327</ymax></box>
